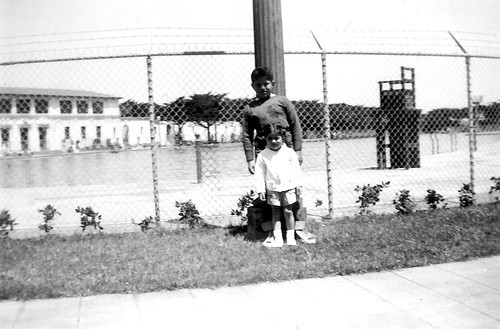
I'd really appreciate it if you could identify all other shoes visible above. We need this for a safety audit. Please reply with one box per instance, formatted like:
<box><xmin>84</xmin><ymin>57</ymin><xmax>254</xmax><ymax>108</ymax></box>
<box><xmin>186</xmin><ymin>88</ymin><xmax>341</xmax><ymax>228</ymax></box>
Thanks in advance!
<box><xmin>271</xmin><ymin>240</ymin><xmax>283</xmax><ymax>247</ymax></box>
<box><xmin>286</xmin><ymin>237</ymin><xmax>297</xmax><ymax>246</ymax></box>
<box><xmin>294</xmin><ymin>229</ymin><xmax>316</xmax><ymax>244</ymax></box>
<box><xmin>262</xmin><ymin>237</ymin><xmax>275</xmax><ymax>247</ymax></box>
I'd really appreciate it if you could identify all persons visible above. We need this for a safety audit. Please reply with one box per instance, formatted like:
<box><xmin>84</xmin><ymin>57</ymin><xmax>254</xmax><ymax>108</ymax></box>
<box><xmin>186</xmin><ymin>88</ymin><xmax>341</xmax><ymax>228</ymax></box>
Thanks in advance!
<box><xmin>254</xmin><ymin>122</ymin><xmax>304</xmax><ymax>246</ymax></box>
<box><xmin>242</xmin><ymin>65</ymin><xmax>317</xmax><ymax>246</ymax></box>
